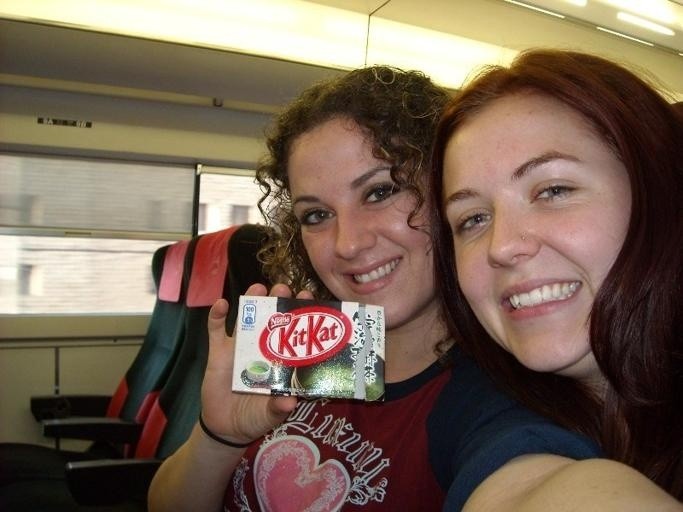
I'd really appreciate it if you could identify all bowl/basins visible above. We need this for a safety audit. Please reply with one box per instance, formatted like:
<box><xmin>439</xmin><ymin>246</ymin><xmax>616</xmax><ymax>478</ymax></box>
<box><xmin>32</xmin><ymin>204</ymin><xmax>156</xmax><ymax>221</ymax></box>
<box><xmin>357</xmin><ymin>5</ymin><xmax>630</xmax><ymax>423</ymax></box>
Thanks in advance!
<box><xmin>245</xmin><ymin>359</ymin><xmax>272</xmax><ymax>384</ymax></box>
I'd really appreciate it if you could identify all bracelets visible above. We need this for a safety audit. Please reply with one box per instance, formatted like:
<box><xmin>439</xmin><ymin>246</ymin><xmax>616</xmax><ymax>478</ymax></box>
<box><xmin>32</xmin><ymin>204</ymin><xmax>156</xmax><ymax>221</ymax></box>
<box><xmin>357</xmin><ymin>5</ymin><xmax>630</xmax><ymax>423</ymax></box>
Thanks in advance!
<box><xmin>198</xmin><ymin>411</ymin><xmax>256</xmax><ymax>449</ymax></box>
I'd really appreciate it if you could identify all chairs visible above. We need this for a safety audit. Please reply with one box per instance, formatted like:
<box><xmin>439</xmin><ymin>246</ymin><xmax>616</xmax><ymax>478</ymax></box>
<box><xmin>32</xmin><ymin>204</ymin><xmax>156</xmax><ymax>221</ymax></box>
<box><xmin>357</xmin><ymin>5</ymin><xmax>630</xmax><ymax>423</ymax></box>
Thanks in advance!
<box><xmin>1</xmin><ymin>223</ymin><xmax>282</xmax><ymax>512</ymax></box>
<box><xmin>1</xmin><ymin>235</ymin><xmax>194</xmax><ymax>512</ymax></box>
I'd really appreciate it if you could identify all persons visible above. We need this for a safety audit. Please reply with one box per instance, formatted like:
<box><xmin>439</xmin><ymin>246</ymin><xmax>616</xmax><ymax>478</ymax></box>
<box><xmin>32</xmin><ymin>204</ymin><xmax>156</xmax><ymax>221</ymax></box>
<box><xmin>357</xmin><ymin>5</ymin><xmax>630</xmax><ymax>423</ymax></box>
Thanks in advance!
<box><xmin>143</xmin><ymin>65</ymin><xmax>459</xmax><ymax>512</ymax></box>
<box><xmin>418</xmin><ymin>44</ymin><xmax>683</xmax><ymax>512</ymax></box>
